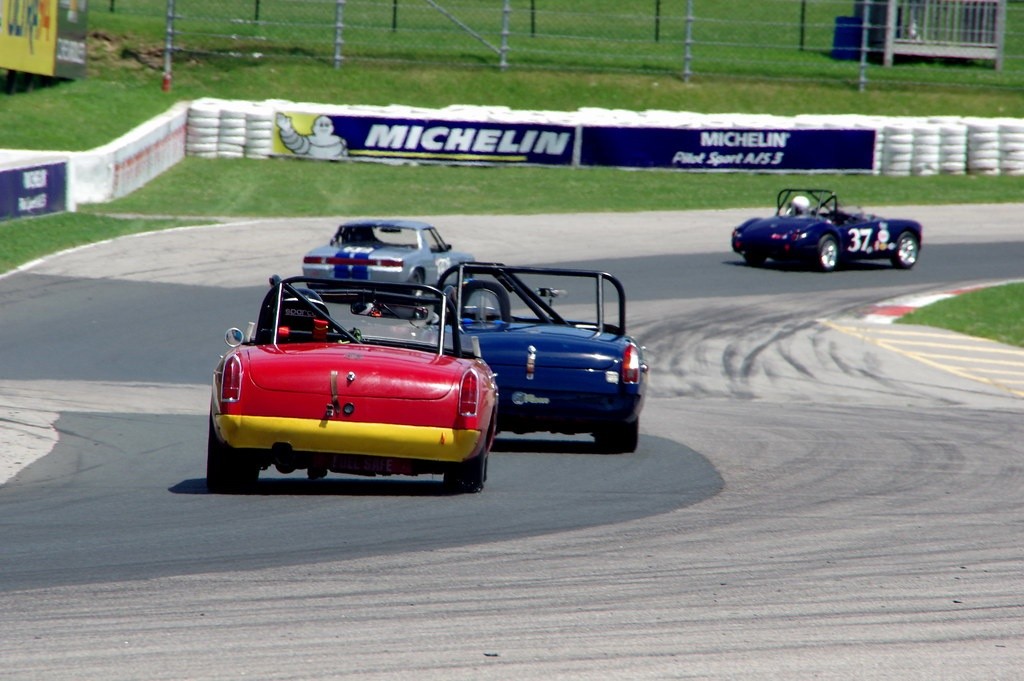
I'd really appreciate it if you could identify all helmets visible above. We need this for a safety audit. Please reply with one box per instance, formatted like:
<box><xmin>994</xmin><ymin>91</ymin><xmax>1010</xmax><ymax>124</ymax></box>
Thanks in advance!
<box><xmin>793</xmin><ymin>196</ymin><xmax>810</xmax><ymax>210</ymax></box>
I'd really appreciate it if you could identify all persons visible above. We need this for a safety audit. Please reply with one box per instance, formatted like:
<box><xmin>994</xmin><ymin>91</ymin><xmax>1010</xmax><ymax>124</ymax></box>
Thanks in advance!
<box><xmin>789</xmin><ymin>195</ymin><xmax>833</xmax><ymax>225</ymax></box>
<box><xmin>284</xmin><ymin>287</ymin><xmax>325</xmax><ymax>302</ymax></box>
<box><xmin>350</xmin><ymin>302</ymin><xmax>382</xmax><ymax>317</ymax></box>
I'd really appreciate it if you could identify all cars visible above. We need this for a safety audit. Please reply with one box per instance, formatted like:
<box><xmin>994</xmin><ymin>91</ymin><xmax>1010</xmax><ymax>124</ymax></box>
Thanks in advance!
<box><xmin>301</xmin><ymin>217</ymin><xmax>475</xmax><ymax>306</ymax></box>
<box><xmin>424</xmin><ymin>259</ymin><xmax>652</xmax><ymax>453</ymax></box>
<box><xmin>730</xmin><ymin>188</ymin><xmax>923</xmax><ymax>272</ymax></box>
<box><xmin>204</xmin><ymin>275</ymin><xmax>501</xmax><ymax>493</ymax></box>
